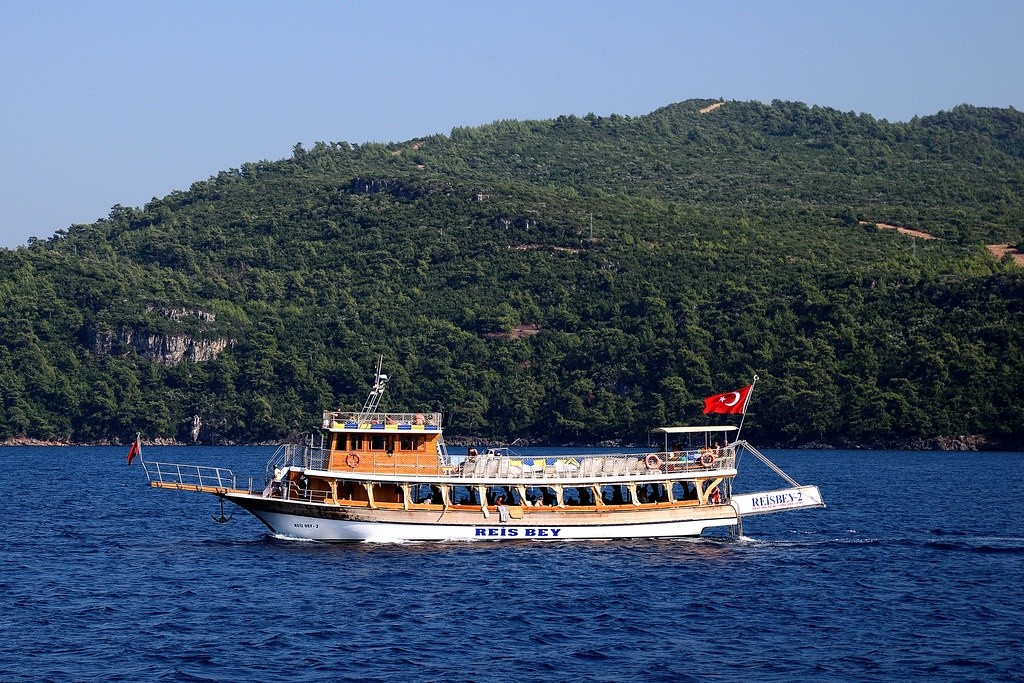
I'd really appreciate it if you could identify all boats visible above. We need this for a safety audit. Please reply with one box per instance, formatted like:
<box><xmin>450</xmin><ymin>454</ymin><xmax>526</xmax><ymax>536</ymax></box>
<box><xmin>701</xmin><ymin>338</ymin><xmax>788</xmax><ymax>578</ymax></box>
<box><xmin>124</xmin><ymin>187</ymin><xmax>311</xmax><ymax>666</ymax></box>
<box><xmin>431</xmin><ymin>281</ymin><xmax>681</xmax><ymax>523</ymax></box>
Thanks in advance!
<box><xmin>142</xmin><ymin>352</ymin><xmax>828</xmax><ymax>540</ymax></box>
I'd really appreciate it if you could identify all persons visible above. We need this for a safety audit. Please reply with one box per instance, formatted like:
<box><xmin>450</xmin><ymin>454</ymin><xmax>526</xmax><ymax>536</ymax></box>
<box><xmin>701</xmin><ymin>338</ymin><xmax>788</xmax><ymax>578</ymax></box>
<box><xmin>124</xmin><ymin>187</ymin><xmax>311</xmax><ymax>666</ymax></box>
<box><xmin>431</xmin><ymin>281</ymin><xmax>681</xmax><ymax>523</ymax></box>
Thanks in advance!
<box><xmin>657</xmin><ymin>440</ymin><xmax>731</xmax><ymax>470</ymax></box>
<box><xmin>470</xmin><ymin>448</ymin><xmax>479</xmax><ymax>462</ymax></box>
<box><xmin>273</xmin><ymin>464</ymin><xmax>282</xmax><ymax>496</ymax></box>
<box><xmin>420</xmin><ymin>483</ymin><xmax>722</xmax><ymax>510</ymax></box>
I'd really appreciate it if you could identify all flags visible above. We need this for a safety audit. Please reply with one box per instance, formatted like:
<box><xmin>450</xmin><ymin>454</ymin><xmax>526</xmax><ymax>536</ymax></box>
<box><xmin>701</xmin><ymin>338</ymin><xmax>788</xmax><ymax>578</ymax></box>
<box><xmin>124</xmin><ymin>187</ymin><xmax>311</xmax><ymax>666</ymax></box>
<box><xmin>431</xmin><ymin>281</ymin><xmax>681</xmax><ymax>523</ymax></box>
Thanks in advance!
<box><xmin>703</xmin><ymin>385</ymin><xmax>752</xmax><ymax>415</ymax></box>
<box><xmin>127</xmin><ymin>437</ymin><xmax>140</xmax><ymax>464</ymax></box>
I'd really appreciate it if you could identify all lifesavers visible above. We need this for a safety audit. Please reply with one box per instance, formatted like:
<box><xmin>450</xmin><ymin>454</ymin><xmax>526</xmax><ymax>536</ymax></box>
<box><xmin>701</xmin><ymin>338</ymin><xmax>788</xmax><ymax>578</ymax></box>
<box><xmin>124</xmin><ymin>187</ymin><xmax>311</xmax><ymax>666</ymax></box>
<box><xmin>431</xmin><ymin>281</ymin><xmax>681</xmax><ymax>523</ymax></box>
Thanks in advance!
<box><xmin>700</xmin><ymin>453</ymin><xmax>714</xmax><ymax>467</ymax></box>
<box><xmin>645</xmin><ymin>454</ymin><xmax>660</xmax><ymax>468</ymax></box>
<box><xmin>346</xmin><ymin>452</ymin><xmax>360</xmax><ymax>468</ymax></box>
